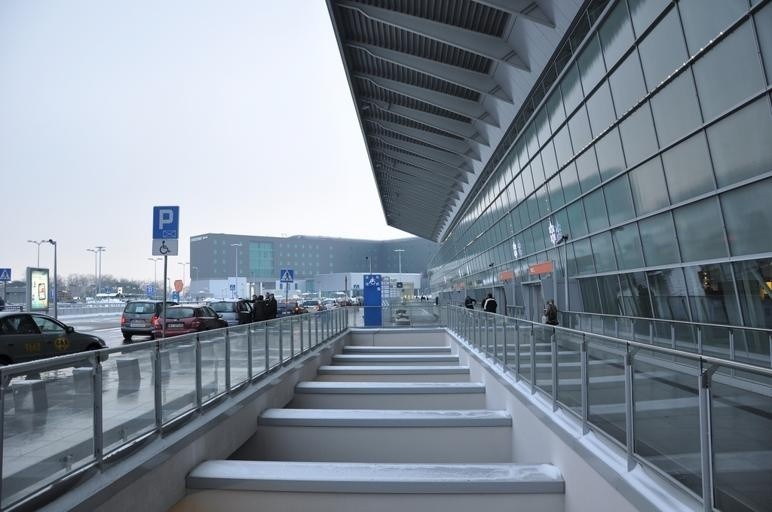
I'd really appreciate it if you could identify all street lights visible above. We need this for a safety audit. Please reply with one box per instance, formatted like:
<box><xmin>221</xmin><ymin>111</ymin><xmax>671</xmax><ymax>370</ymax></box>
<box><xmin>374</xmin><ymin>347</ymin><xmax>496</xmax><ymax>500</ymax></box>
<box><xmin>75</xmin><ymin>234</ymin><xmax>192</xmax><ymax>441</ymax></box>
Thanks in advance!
<box><xmin>27</xmin><ymin>239</ymin><xmax>48</xmax><ymax>269</ymax></box>
<box><xmin>166</xmin><ymin>277</ymin><xmax>171</xmax><ymax>293</ymax></box>
<box><xmin>394</xmin><ymin>249</ymin><xmax>406</xmax><ymax>273</ymax></box>
<box><xmin>177</xmin><ymin>262</ymin><xmax>191</xmax><ymax>292</ymax></box>
<box><xmin>148</xmin><ymin>257</ymin><xmax>164</xmax><ymax>292</ymax></box>
<box><xmin>86</xmin><ymin>248</ymin><xmax>105</xmax><ymax>285</ymax></box>
<box><xmin>231</xmin><ymin>244</ymin><xmax>242</xmax><ymax>299</ymax></box>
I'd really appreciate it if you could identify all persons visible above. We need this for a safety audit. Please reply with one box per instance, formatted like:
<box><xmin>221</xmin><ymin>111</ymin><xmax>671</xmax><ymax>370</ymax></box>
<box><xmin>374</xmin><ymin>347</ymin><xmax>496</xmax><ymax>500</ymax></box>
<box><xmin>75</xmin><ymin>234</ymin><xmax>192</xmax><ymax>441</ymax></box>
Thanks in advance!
<box><xmin>544</xmin><ymin>299</ymin><xmax>559</xmax><ymax>325</ymax></box>
<box><xmin>238</xmin><ymin>292</ymin><xmax>277</xmax><ymax>325</ymax></box>
<box><xmin>464</xmin><ymin>295</ymin><xmax>477</xmax><ymax>309</ymax></box>
<box><xmin>484</xmin><ymin>293</ymin><xmax>498</xmax><ymax>313</ymax></box>
<box><xmin>636</xmin><ymin>282</ymin><xmax>657</xmax><ymax>319</ymax></box>
<box><xmin>481</xmin><ymin>297</ymin><xmax>488</xmax><ymax>309</ymax></box>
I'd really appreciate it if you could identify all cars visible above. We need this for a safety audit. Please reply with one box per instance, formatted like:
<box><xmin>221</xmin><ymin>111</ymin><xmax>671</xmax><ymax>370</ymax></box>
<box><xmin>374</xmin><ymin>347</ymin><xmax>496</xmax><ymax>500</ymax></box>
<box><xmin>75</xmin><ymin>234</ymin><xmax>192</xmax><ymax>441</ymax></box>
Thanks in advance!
<box><xmin>119</xmin><ymin>299</ymin><xmax>180</xmax><ymax>341</ymax></box>
<box><xmin>300</xmin><ymin>300</ymin><xmax>327</xmax><ymax>312</ymax></box>
<box><xmin>0</xmin><ymin>309</ymin><xmax>109</xmax><ymax>388</ymax></box>
<box><xmin>152</xmin><ymin>304</ymin><xmax>230</xmax><ymax>339</ymax></box>
<box><xmin>278</xmin><ymin>302</ymin><xmax>308</xmax><ymax>317</ymax></box>
<box><xmin>324</xmin><ymin>297</ymin><xmax>365</xmax><ymax>310</ymax></box>
<box><xmin>207</xmin><ymin>300</ymin><xmax>256</xmax><ymax>325</ymax></box>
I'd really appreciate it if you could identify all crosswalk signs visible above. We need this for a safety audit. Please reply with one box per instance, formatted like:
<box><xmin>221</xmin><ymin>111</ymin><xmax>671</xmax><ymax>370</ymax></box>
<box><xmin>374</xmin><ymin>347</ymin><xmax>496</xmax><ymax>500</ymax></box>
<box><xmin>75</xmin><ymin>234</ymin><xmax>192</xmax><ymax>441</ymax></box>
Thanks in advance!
<box><xmin>1</xmin><ymin>269</ymin><xmax>12</xmax><ymax>281</ymax></box>
<box><xmin>280</xmin><ymin>270</ymin><xmax>294</xmax><ymax>283</ymax></box>
